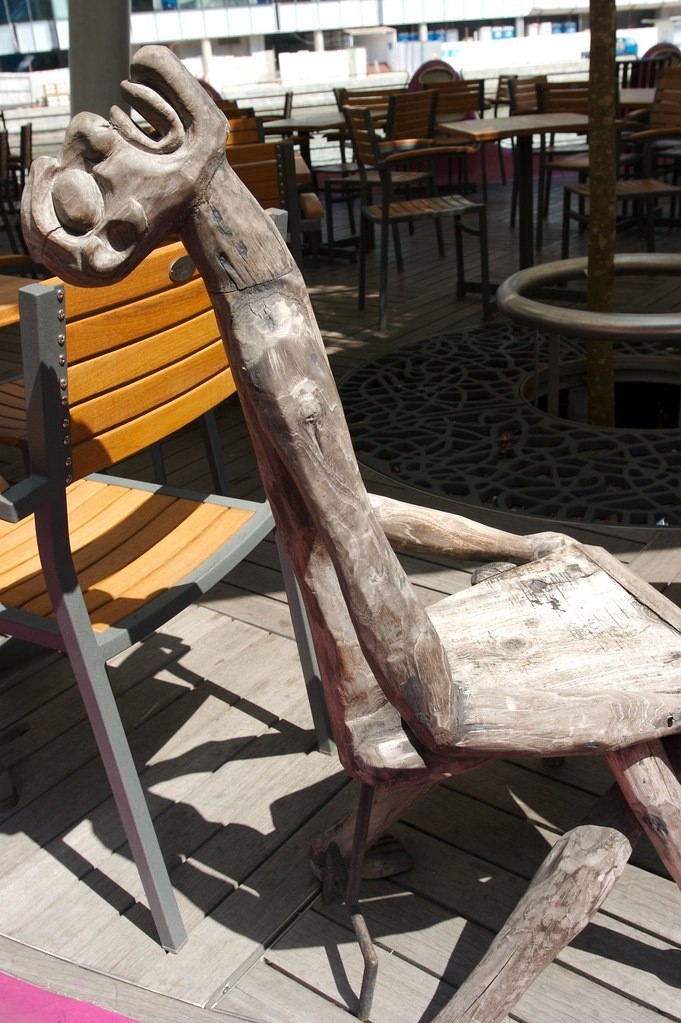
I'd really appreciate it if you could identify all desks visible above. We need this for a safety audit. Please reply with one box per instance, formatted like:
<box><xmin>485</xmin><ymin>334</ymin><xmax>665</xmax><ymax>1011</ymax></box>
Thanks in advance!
<box><xmin>263</xmin><ymin>110</ymin><xmax>389</xmax><ymax>263</ymax></box>
<box><xmin>438</xmin><ymin>113</ymin><xmax>587</xmax><ymax>311</ymax></box>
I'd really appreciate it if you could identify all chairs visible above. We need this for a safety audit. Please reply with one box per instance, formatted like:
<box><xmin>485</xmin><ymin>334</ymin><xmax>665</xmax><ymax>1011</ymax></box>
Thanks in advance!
<box><xmin>0</xmin><ymin>43</ymin><xmax>681</xmax><ymax>1021</ymax></box>
